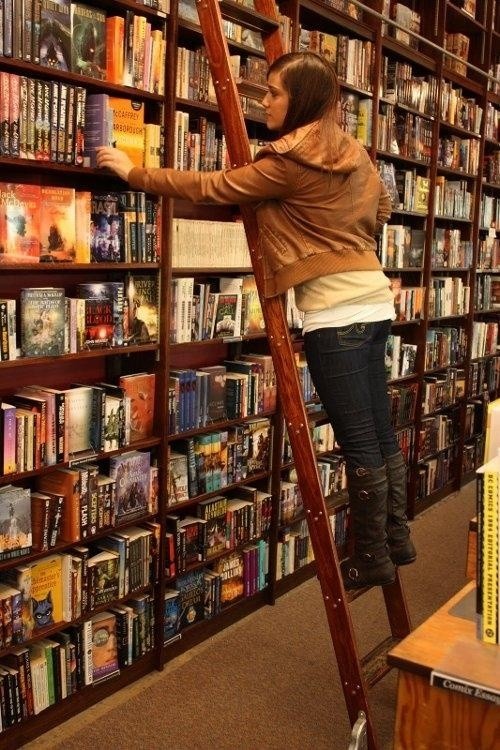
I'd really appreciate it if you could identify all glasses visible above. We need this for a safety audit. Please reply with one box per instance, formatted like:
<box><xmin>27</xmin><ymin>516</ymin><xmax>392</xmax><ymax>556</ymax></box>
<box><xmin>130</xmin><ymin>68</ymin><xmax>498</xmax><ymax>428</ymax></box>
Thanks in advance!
<box><xmin>92</xmin><ymin>625</ymin><xmax>117</xmax><ymax>648</ymax></box>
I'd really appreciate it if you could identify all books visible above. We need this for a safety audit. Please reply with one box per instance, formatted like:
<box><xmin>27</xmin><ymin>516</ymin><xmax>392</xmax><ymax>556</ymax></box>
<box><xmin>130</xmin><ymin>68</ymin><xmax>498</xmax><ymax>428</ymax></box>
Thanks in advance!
<box><xmin>388</xmin><ymin>356</ymin><xmax>500</xmax><ymax>499</ymax></box>
<box><xmin>2</xmin><ymin>1</ymin><xmax>278</xmax><ymax>172</ymax></box>
<box><xmin>296</xmin><ymin>1</ymin><xmax>498</xmax><ymax>228</ymax></box>
<box><xmin>1</xmin><ymin>183</ymin><xmax>266</xmax><ymax>362</ymax></box>
<box><xmin>276</xmin><ymin>352</ymin><xmax>352</xmax><ymax>581</ymax></box>
<box><xmin>474</xmin><ymin>394</ymin><xmax>499</xmax><ymax>646</ymax></box>
<box><xmin>3</xmin><ymin>353</ymin><xmax>276</xmax><ymax>732</ymax></box>
<box><xmin>374</xmin><ymin>222</ymin><xmax>500</xmax><ymax>381</ymax></box>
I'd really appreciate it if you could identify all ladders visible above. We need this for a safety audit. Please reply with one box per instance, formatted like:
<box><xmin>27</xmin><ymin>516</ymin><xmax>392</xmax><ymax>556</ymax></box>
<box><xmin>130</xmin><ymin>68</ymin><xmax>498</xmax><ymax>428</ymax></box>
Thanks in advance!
<box><xmin>195</xmin><ymin>0</ymin><xmax>418</xmax><ymax>750</ymax></box>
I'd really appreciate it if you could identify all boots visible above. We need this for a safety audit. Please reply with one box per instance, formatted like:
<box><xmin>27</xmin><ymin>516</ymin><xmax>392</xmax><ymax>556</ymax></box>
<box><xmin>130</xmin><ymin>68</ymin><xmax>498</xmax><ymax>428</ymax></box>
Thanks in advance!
<box><xmin>383</xmin><ymin>448</ymin><xmax>417</xmax><ymax>566</ymax></box>
<box><xmin>339</xmin><ymin>464</ymin><xmax>396</xmax><ymax>591</ymax></box>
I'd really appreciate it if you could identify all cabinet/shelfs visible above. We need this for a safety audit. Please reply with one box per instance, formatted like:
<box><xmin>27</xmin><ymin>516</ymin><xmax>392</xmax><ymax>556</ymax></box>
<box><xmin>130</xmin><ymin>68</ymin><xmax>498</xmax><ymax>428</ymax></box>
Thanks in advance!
<box><xmin>0</xmin><ymin>1</ymin><xmax>499</xmax><ymax>750</ymax></box>
